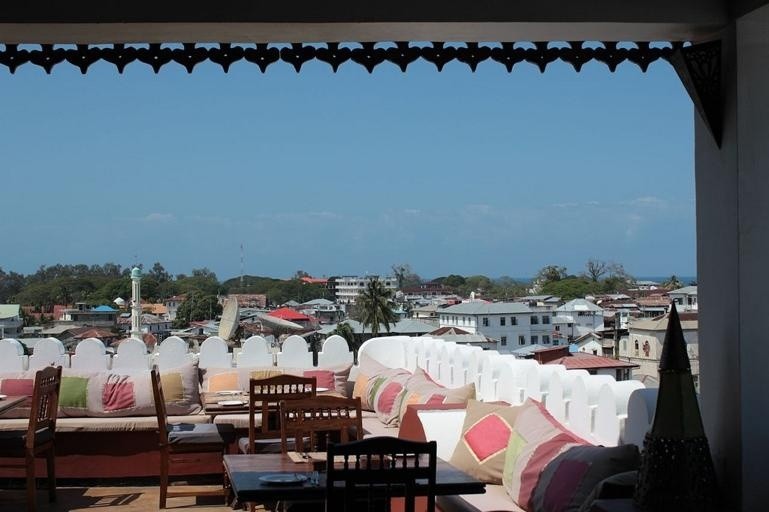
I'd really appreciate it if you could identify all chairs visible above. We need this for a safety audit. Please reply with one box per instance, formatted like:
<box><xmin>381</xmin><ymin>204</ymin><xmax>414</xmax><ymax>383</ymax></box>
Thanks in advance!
<box><xmin>280</xmin><ymin>394</ymin><xmax>437</xmax><ymax>512</ymax></box>
<box><xmin>0</xmin><ymin>365</ymin><xmax>64</xmax><ymax>510</ymax></box>
<box><xmin>239</xmin><ymin>374</ymin><xmax>317</xmax><ymax>492</ymax></box>
<box><xmin>151</xmin><ymin>365</ymin><xmax>239</xmax><ymax>509</ymax></box>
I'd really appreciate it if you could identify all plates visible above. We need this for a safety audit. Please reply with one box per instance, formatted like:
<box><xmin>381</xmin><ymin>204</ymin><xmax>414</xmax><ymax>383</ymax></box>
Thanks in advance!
<box><xmin>305</xmin><ymin>388</ymin><xmax>328</xmax><ymax>392</ymax></box>
<box><xmin>259</xmin><ymin>474</ymin><xmax>308</xmax><ymax>483</ymax></box>
<box><xmin>216</xmin><ymin>400</ymin><xmax>247</xmax><ymax>406</ymax></box>
<box><xmin>217</xmin><ymin>390</ymin><xmax>242</xmax><ymax>395</ymax></box>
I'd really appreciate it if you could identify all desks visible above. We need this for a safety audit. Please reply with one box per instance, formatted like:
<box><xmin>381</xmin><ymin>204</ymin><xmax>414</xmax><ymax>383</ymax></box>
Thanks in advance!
<box><xmin>0</xmin><ymin>394</ymin><xmax>28</xmax><ymax>418</ymax></box>
<box><xmin>205</xmin><ymin>403</ymin><xmax>277</xmax><ymax>424</ymax></box>
<box><xmin>222</xmin><ymin>454</ymin><xmax>486</xmax><ymax>512</ymax></box>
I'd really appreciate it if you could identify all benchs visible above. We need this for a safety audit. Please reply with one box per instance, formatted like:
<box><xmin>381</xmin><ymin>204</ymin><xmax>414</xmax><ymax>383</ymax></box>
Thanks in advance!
<box><xmin>0</xmin><ymin>324</ymin><xmax>357</xmax><ymax>482</ymax></box>
<box><xmin>343</xmin><ymin>333</ymin><xmax>664</xmax><ymax>511</ymax></box>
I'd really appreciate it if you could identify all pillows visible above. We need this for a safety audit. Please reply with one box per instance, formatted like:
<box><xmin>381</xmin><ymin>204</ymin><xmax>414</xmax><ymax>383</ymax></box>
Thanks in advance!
<box><xmin>199</xmin><ymin>366</ymin><xmax>243</xmax><ymax>392</ymax></box>
<box><xmin>349</xmin><ymin>346</ymin><xmax>416</xmax><ymax>426</ymax></box>
<box><xmin>55</xmin><ymin>364</ymin><xmax>203</xmax><ymax>419</ymax></box>
<box><xmin>0</xmin><ymin>370</ymin><xmax>45</xmax><ymax>420</ymax></box>
<box><xmin>238</xmin><ymin>362</ymin><xmax>353</xmax><ymax>397</ymax></box>
<box><xmin>498</xmin><ymin>392</ymin><xmax>642</xmax><ymax>511</ymax></box>
<box><xmin>443</xmin><ymin>389</ymin><xmax>528</xmax><ymax>487</ymax></box>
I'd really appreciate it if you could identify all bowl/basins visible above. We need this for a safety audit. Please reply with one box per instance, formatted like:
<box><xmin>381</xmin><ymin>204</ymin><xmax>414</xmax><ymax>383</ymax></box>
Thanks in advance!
<box><xmin>360</xmin><ymin>456</ymin><xmax>396</xmax><ymax>469</ymax></box>
